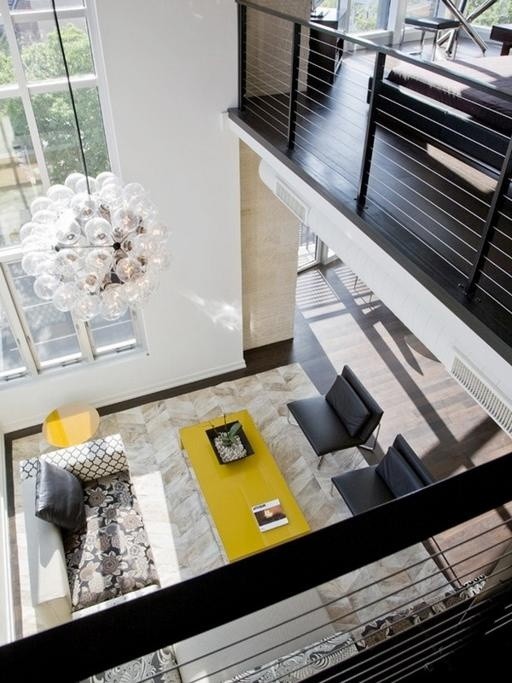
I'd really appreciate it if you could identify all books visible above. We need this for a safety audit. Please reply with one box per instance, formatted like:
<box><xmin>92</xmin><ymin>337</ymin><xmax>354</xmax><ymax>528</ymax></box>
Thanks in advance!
<box><xmin>252</xmin><ymin>498</ymin><xmax>289</xmax><ymax>533</ymax></box>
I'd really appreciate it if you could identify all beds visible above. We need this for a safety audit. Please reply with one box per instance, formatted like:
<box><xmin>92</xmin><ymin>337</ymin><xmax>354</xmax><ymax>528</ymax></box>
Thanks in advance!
<box><xmin>367</xmin><ymin>56</ymin><xmax>512</xmax><ymax>185</ymax></box>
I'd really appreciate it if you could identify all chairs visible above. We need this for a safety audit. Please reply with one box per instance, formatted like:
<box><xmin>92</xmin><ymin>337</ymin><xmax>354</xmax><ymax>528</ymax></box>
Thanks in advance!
<box><xmin>286</xmin><ymin>365</ymin><xmax>383</xmax><ymax>471</ymax></box>
<box><xmin>330</xmin><ymin>434</ymin><xmax>437</xmax><ymax>515</ymax></box>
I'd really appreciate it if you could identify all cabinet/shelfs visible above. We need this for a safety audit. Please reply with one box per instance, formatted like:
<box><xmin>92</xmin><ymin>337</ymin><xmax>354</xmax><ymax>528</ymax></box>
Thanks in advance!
<box><xmin>308</xmin><ymin>9</ymin><xmax>344</xmax><ymax>85</ymax></box>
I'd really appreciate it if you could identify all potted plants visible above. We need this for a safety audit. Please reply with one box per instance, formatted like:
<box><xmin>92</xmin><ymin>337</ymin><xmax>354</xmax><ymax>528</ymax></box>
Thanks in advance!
<box><xmin>205</xmin><ymin>413</ymin><xmax>255</xmax><ymax>464</ymax></box>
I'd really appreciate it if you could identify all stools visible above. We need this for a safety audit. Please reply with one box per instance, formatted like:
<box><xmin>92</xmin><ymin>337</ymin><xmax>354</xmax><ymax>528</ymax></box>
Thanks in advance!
<box><xmin>398</xmin><ymin>16</ymin><xmax>463</xmax><ymax>64</ymax></box>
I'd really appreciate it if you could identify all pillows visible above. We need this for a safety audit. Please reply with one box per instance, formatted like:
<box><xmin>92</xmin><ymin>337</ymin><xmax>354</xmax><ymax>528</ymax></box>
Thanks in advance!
<box><xmin>374</xmin><ymin>447</ymin><xmax>423</xmax><ymax>498</ymax></box>
<box><xmin>36</xmin><ymin>458</ymin><xmax>86</xmax><ymax>531</ymax></box>
<box><xmin>325</xmin><ymin>375</ymin><xmax>369</xmax><ymax>438</ymax></box>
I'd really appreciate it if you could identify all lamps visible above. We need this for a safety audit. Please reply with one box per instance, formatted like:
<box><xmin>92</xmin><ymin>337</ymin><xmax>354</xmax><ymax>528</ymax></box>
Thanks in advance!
<box><xmin>18</xmin><ymin>1</ymin><xmax>166</xmax><ymax>320</ymax></box>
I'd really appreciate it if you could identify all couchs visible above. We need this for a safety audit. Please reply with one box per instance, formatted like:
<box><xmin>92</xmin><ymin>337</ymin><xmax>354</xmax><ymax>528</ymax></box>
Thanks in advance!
<box><xmin>223</xmin><ymin>573</ymin><xmax>489</xmax><ymax>682</ymax></box>
<box><xmin>19</xmin><ymin>433</ymin><xmax>182</xmax><ymax>683</ymax></box>
